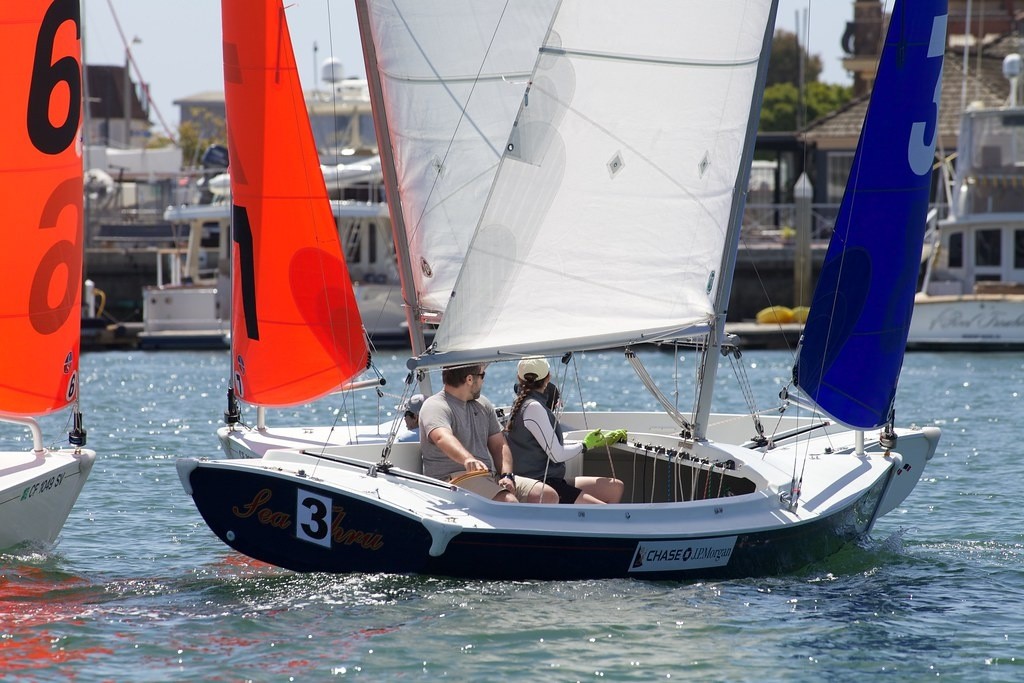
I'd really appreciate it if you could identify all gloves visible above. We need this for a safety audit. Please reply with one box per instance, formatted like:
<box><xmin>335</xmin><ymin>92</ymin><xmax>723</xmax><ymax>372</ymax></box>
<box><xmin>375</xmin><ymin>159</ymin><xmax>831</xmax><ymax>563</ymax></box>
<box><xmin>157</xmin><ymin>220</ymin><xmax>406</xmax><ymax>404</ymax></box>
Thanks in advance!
<box><xmin>606</xmin><ymin>429</ymin><xmax>628</xmax><ymax>445</ymax></box>
<box><xmin>584</xmin><ymin>428</ymin><xmax>606</xmax><ymax>448</ymax></box>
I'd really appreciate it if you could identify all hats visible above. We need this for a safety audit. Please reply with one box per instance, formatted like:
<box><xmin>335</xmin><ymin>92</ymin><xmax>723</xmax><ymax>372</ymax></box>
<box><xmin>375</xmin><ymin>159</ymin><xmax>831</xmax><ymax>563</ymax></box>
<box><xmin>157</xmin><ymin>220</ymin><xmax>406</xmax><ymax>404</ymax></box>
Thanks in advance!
<box><xmin>518</xmin><ymin>355</ymin><xmax>550</xmax><ymax>382</ymax></box>
<box><xmin>394</xmin><ymin>394</ymin><xmax>428</xmax><ymax>415</ymax></box>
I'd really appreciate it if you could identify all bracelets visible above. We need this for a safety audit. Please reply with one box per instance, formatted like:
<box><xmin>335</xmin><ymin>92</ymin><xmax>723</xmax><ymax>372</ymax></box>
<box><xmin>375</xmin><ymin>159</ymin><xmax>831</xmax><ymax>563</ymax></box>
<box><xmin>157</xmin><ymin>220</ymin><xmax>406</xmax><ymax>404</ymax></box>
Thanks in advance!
<box><xmin>500</xmin><ymin>473</ymin><xmax>512</xmax><ymax>479</ymax></box>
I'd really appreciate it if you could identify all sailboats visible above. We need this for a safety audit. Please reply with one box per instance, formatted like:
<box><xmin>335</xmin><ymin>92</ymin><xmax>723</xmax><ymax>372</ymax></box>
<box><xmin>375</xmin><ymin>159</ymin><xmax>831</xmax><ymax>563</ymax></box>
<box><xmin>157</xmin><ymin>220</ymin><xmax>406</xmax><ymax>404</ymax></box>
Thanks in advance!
<box><xmin>175</xmin><ymin>2</ymin><xmax>953</xmax><ymax>582</ymax></box>
<box><xmin>0</xmin><ymin>1</ymin><xmax>97</xmax><ymax>556</ymax></box>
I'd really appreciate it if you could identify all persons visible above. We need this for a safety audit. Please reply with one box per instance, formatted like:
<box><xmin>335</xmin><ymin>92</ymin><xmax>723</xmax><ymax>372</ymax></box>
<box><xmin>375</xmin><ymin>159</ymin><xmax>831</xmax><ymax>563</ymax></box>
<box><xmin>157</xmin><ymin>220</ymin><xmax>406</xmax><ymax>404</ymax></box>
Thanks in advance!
<box><xmin>418</xmin><ymin>363</ymin><xmax>559</xmax><ymax>503</ymax></box>
<box><xmin>503</xmin><ymin>355</ymin><xmax>627</xmax><ymax>505</ymax></box>
<box><xmin>394</xmin><ymin>394</ymin><xmax>428</xmax><ymax>442</ymax></box>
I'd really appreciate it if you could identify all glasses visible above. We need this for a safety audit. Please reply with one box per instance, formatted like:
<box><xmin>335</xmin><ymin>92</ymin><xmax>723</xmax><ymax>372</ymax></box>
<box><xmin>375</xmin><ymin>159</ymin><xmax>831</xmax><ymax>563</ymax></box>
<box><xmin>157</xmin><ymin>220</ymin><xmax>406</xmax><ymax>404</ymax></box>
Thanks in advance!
<box><xmin>466</xmin><ymin>371</ymin><xmax>485</xmax><ymax>379</ymax></box>
<box><xmin>404</xmin><ymin>413</ymin><xmax>414</xmax><ymax>417</ymax></box>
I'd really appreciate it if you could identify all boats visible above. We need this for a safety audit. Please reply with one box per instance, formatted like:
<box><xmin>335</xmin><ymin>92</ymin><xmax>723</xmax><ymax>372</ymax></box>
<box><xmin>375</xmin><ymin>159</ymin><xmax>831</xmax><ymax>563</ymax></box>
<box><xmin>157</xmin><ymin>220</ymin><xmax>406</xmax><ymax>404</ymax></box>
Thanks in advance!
<box><xmin>84</xmin><ymin>1</ymin><xmax>1023</xmax><ymax>354</ymax></box>
